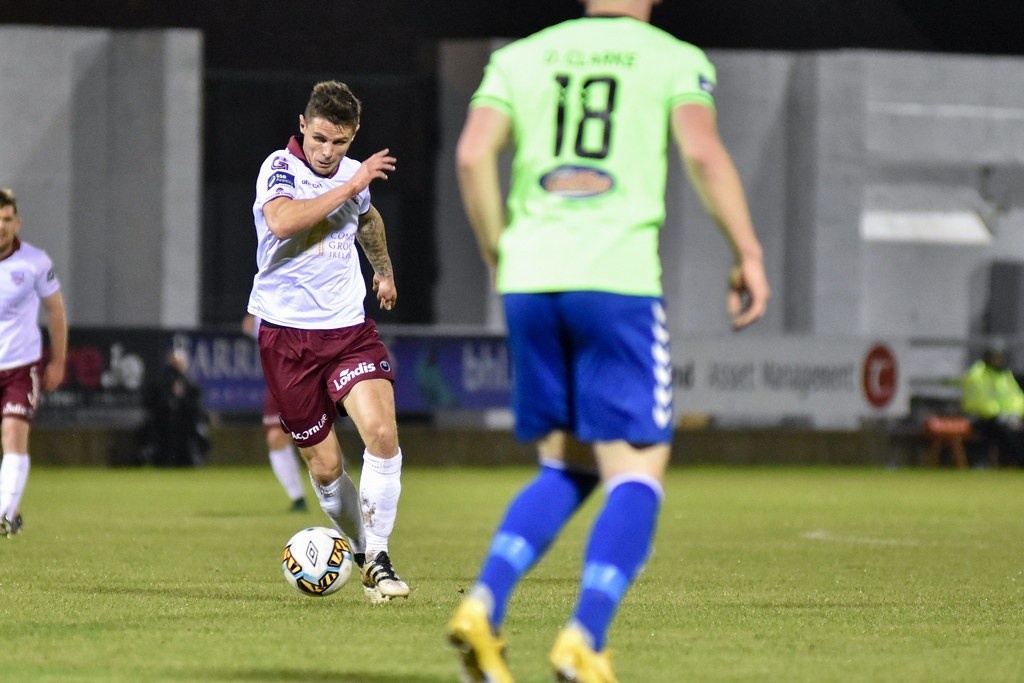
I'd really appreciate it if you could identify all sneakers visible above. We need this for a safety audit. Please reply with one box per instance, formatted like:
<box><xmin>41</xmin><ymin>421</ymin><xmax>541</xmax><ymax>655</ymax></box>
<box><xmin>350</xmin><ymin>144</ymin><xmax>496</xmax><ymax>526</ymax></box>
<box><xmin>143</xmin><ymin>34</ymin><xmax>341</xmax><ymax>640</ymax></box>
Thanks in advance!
<box><xmin>362</xmin><ymin>550</ymin><xmax>410</xmax><ymax>599</ymax></box>
<box><xmin>354</xmin><ymin>552</ymin><xmax>389</xmax><ymax>604</ymax></box>
<box><xmin>445</xmin><ymin>596</ymin><xmax>516</xmax><ymax>683</ymax></box>
<box><xmin>549</xmin><ymin>624</ymin><xmax>619</xmax><ymax>683</ymax></box>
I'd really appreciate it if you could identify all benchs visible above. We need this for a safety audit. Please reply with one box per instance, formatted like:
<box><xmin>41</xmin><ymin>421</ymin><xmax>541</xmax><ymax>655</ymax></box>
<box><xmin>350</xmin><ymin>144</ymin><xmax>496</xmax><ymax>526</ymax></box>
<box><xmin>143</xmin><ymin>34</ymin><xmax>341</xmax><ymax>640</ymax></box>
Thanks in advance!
<box><xmin>925</xmin><ymin>415</ymin><xmax>999</xmax><ymax>470</ymax></box>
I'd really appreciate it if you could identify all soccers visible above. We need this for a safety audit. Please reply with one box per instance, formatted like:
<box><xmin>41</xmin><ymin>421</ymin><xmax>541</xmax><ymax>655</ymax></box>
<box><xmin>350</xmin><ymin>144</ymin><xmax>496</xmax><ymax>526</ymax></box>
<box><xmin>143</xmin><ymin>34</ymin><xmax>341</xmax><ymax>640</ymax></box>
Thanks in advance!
<box><xmin>282</xmin><ymin>525</ymin><xmax>354</xmax><ymax>596</ymax></box>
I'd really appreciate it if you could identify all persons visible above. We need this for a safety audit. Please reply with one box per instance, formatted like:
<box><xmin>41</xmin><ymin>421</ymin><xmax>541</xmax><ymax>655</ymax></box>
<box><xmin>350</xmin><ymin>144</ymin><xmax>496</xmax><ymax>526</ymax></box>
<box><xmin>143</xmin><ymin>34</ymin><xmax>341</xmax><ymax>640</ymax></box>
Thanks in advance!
<box><xmin>0</xmin><ymin>191</ymin><xmax>64</xmax><ymax>538</ymax></box>
<box><xmin>966</xmin><ymin>338</ymin><xmax>1022</xmax><ymax>468</ymax></box>
<box><xmin>444</xmin><ymin>0</ymin><xmax>774</xmax><ymax>682</ymax></box>
<box><xmin>241</xmin><ymin>81</ymin><xmax>408</xmax><ymax>608</ymax></box>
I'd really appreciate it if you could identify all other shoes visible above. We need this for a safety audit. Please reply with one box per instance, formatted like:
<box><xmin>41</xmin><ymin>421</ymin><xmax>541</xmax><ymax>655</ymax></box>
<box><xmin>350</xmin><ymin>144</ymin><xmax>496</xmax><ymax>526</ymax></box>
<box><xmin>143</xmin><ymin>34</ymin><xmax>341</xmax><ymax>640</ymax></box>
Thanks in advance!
<box><xmin>0</xmin><ymin>513</ymin><xmax>22</xmax><ymax>537</ymax></box>
<box><xmin>292</xmin><ymin>499</ymin><xmax>305</xmax><ymax>512</ymax></box>
